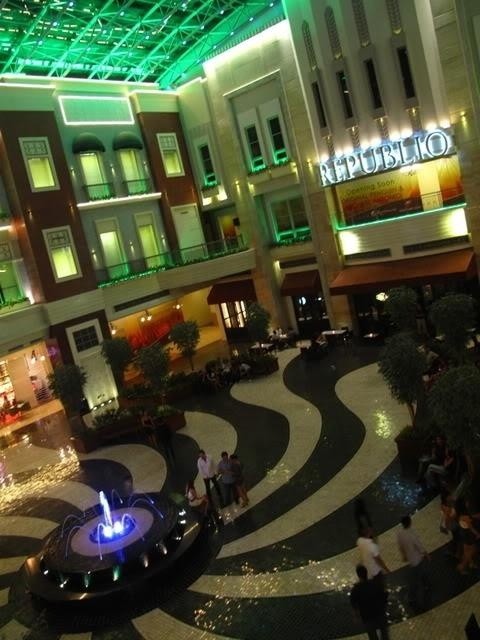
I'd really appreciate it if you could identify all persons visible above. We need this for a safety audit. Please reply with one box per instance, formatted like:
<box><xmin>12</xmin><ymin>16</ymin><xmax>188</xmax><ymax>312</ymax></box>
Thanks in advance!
<box><xmin>141</xmin><ymin>410</ymin><xmax>159</xmax><ymax>451</ymax></box>
<box><xmin>206</xmin><ymin>358</ymin><xmax>253</xmax><ymax>391</ymax></box>
<box><xmin>423</xmin><ymin>346</ymin><xmax>438</xmax><ymax>369</ymax></box>
<box><xmin>416</xmin><ymin>303</ymin><xmax>428</xmax><ymax>336</ymax></box>
<box><xmin>183</xmin><ymin>451</ymin><xmax>249</xmax><ymax>530</ymax></box>
<box><xmin>350</xmin><ymin>515</ymin><xmax>434</xmax><ymax>638</ymax></box>
<box><xmin>272</xmin><ymin>326</ymin><xmax>297</xmax><ymax>349</ymax></box>
<box><xmin>413</xmin><ymin>433</ymin><xmax>480</xmax><ymax>575</ymax></box>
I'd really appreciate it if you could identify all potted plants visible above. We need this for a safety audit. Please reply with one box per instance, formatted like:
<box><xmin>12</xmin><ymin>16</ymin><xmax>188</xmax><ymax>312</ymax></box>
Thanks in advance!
<box><xmin>377</xmin><ymin>327</ymin><xmax>437</xmax><ymax>480</ymax></box>
<box><xmin>46</xmin><ymin>301</ymin><xmax>280</xmax><ymax>453</ymax></box>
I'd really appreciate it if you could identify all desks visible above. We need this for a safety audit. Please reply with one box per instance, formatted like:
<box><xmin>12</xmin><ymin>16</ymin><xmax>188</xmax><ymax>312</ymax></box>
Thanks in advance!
<box><xmin>322</xmin><ymin>330</ymin><xmax>348</xmax><ymax>346</ymax></box>
<box><xmin>279</xmin><ymin>333</ymin><xmax>288</xmax><ymax>348</ymax></box>
